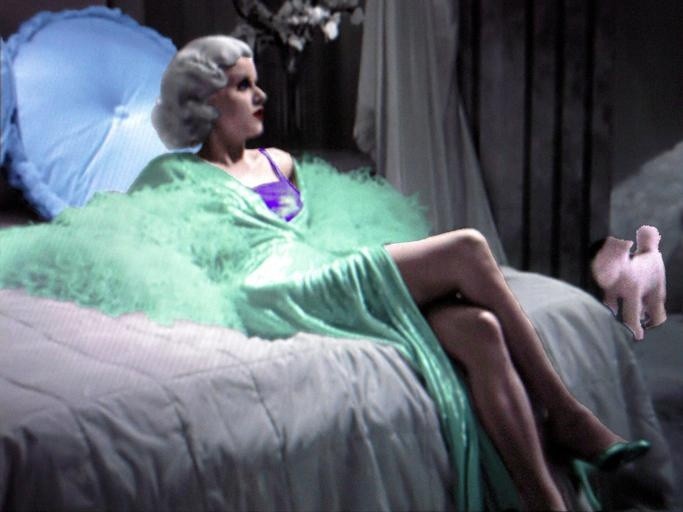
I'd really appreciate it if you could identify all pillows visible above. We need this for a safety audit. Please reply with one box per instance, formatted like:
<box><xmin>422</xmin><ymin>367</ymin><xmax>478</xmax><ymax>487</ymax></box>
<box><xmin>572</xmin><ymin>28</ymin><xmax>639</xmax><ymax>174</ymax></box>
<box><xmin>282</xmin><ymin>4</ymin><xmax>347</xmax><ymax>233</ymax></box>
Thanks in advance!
<box><xmin>0</xmin><ymin>6</ymin><xmax>193</xmax><ymax>217</ymax></box>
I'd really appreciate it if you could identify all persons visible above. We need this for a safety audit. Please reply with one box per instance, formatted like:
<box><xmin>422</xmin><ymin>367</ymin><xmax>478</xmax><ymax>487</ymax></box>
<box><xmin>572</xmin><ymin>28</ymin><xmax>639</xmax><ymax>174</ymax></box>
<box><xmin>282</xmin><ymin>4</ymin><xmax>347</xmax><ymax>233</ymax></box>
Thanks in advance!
<box><xmin>127</xmin><ymin>34</ymin><xmax>653</xmax><ymax>512</ymax></box>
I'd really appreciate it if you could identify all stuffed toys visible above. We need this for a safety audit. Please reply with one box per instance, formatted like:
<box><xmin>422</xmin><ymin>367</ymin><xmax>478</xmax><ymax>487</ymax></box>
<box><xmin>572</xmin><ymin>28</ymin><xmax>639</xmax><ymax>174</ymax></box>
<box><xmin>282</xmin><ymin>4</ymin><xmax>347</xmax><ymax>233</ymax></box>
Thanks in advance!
<box><xmin>591</xmin><ymin>225</ymin><xmax>666</xmax><ymax>341</ymax></box>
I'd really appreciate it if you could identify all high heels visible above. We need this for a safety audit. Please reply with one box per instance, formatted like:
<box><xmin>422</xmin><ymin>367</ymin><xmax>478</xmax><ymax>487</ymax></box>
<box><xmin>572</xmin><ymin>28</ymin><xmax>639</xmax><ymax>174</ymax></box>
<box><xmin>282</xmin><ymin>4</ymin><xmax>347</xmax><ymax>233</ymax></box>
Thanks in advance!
<box><xmin>565</xmin><ymin>436</ymin><xmax>649</xmax><ymax>511</ymax></box>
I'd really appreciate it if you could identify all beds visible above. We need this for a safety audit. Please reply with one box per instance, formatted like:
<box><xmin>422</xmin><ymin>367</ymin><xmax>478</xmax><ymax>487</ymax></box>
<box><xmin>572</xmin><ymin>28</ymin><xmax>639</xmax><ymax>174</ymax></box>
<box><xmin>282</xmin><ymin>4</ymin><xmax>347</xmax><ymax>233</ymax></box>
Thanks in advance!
<box><xmin>0</xmin><ymin>206</ymin><xmax>633</xmax><ymax>511</ymax></box>
